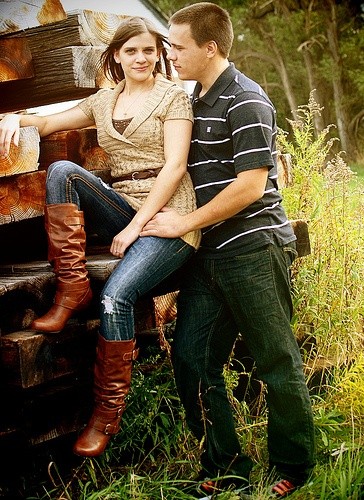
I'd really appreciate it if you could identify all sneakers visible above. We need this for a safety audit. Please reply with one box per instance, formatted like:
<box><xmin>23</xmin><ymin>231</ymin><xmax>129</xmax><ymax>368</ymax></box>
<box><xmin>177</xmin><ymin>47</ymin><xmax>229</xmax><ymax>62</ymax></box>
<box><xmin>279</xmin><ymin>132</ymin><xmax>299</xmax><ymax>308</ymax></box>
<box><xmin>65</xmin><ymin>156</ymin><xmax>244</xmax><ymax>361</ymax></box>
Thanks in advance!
<box><xmin>196</xmin><ymin>478</ymin><xmax>222</xmax><ymax>496</ymax></box>
<box><xmin>272</xmin><ymin>479</ymin><xmax>296</xmax><ymax>498</ymax></box>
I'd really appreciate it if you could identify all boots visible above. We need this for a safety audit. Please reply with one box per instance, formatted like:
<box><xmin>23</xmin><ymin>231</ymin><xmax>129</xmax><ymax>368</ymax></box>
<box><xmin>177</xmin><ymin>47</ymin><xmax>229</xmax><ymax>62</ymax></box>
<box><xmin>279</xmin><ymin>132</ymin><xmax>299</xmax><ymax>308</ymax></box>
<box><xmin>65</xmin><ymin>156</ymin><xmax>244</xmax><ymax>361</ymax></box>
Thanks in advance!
<box><xmin>72</xmin><ymin>330</ymin><xmax>139</xmax><ymax>456</ymax></box>
<box><xmin>32</xmin><ymin>203</ymin><xmax>92</xmax><ymax>334</ymax></box>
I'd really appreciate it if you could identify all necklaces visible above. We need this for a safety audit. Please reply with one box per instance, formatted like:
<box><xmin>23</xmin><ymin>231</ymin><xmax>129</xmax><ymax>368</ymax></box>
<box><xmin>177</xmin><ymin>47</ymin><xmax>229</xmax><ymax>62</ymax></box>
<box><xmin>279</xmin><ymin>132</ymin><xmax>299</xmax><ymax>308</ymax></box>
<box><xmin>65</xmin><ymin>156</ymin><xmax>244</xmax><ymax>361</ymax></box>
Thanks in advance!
<box><xmin>121</xmin><ymin>87</ymin><xmax>149</xmax><ymax>117</ymax></box>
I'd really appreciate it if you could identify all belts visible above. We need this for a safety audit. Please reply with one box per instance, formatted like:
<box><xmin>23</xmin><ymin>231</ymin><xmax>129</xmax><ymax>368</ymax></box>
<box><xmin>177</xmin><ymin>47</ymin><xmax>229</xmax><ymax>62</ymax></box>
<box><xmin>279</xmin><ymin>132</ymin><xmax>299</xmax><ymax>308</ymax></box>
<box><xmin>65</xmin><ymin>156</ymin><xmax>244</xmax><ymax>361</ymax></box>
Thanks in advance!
<box><xmin>111</xmin><ymin>167</ymin><xmax>163</xmax><ymax>184</ymax></box>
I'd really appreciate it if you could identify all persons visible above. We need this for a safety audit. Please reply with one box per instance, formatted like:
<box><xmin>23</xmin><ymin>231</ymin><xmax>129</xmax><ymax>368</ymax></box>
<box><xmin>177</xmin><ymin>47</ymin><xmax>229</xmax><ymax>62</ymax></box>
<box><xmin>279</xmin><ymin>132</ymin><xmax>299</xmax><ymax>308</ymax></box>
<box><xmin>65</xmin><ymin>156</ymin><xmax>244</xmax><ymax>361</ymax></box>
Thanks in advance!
<box><xmin>137</xmin><ymin>2</ymin><xmax>314</xmax><ymax>500</ymax></box>
<box><xmin>0</xmin><ymin>16</ymin><xmax>203</xmax><ymax>457</ymax></box>
